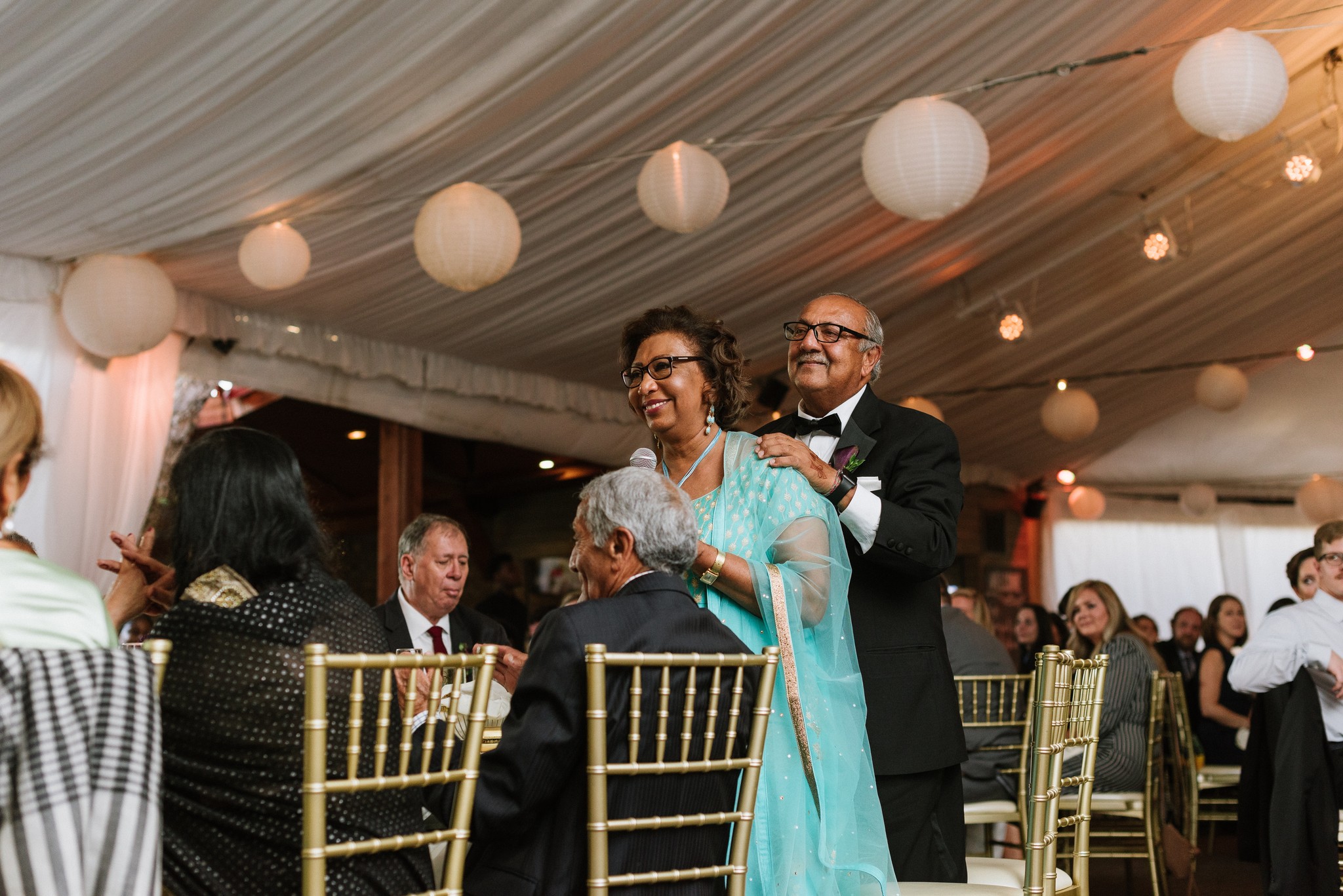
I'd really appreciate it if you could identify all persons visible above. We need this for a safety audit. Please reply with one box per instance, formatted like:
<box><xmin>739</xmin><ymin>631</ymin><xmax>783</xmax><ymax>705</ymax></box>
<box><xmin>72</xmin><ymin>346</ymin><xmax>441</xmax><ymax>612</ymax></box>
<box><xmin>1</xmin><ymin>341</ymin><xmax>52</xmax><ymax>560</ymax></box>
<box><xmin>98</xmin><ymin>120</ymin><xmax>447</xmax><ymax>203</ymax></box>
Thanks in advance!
<box><xmin>0</xmin><ymin>363</ymin><xmax>120</xmax><ymax>650</ymax></box>
<box><xmin>98</xmin><ymin>427</ymin><xmax>436</xmax><ymax>896</ymax></box>
<box><xmin>387</xmin><ymin>465</ymin><xmax>761</xmax><ymax>896</ymax></box>
<box><xmin>371</xmin><ymin>512</ymin><xmax>512</xmax><ymax>686</ymax></box>
<box><xmin>740</xmin><ymin>292</ymin><xmax>967</xmax><ymax>882</ymax></box>
<box><xmin>480</xmin><ymin>562</ymin><xmax>581</xmax><ymax>654</ymax></box>
<box><xmin>623</xmin><ymin>307</ymin><xmax>900</xmax><ymax>896</ymax></box>
<box><xmin>940</xmin><ymin>521</ymin><xmax>1343</xmax><ymax>896</ymax></box>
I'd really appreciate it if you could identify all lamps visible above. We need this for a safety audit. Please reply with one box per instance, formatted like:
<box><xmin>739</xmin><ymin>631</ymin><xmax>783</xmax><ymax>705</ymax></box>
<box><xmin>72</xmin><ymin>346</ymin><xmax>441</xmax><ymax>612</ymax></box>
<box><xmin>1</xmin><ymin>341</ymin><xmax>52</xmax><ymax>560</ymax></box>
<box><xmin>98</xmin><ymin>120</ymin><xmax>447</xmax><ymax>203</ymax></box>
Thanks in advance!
<box><xmin>1143</xmin><ymin>225</ymin><xmax>1169</xmax><ymax>262</ymax></box>
<box><xmin>1281</xmin><ymin>139</ymin><xmax>1322</xmax><ymax>183</ymax></box>
<box><xmin>995</xmin><ymin>306</ymin><xmax>1025</xmax><ymax>342</ymax></box>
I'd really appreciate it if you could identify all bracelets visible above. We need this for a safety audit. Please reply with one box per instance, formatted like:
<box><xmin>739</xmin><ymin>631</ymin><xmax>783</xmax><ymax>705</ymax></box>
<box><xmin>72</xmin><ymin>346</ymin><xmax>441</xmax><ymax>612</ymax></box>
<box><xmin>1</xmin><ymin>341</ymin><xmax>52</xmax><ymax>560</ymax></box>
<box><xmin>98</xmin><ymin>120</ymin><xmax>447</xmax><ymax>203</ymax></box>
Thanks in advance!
<box><xmin>822</xmin><ymin>469</ymin><xmax>842</xmax><ymax>497</ymax></box>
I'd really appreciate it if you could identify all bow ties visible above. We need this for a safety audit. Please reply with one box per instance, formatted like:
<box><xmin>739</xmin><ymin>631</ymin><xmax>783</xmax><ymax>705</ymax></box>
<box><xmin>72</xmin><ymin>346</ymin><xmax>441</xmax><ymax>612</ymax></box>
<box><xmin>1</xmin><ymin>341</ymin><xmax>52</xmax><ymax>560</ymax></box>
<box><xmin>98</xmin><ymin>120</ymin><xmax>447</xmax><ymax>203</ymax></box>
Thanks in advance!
<box><xmin>792</xmin><ymin>412</ymin><xmax>842</xmax><ymax>439</ymax></box>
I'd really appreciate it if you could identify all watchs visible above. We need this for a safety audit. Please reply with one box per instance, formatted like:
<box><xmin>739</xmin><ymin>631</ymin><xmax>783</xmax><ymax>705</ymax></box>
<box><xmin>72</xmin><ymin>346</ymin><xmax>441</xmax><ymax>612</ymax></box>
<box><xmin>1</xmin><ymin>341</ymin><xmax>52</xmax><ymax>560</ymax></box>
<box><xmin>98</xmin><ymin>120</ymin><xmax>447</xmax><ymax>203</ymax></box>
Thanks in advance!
<box><xmin>825</xmin><ymin>468</ymin><xmax>858</xmax><ymax>505</ymax></box>
<box><xmin>700</xmin><ymin>548</ymin><xmax>726</xmax><ymax>585</ymax></box>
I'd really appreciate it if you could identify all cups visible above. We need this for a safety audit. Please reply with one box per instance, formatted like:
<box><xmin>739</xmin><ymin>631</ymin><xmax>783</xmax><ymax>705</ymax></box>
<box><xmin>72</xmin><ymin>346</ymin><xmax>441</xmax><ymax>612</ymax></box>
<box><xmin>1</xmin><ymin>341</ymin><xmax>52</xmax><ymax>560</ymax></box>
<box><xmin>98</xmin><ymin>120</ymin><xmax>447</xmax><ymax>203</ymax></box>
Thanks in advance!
<box><xmin>439</xmin><ymin>667</ymin><xmax>466</xmax><ymax>714</ymax></box>
<box><xmin>395</xmin><ymin>648</ymin><xmax>424</xmax><ymax>673</ymax></box>
<box><xmin>472</xmin><ymin>667</ymin><xmax>497</xmax><ymax>682</ymax></box>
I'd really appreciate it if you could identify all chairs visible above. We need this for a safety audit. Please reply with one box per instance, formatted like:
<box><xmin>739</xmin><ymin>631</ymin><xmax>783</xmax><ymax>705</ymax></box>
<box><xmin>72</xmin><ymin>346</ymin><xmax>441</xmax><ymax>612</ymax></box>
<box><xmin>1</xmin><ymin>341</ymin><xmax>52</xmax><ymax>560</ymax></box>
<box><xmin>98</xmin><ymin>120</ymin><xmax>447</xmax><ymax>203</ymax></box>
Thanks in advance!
<box><xmin>583</xmin><ymin>643</ymin><xmax>782</xmax><ymax>896</ymax></box>
<box><xmin>300</xmin><ymin>644</ymin><xmax>498</xmax><ymax>896</ymax></box>
<box><xmin>862</xmin><ymin>644</ymin><xmax>1240</xmax><ymax>896</ymax></box>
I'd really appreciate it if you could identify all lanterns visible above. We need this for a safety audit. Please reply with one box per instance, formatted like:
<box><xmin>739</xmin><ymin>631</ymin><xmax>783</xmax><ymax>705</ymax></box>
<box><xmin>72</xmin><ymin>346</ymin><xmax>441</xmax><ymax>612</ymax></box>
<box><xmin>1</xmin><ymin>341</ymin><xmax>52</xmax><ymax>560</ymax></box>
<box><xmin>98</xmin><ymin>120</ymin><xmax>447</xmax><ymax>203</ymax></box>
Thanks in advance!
<box><xmin>62</xmin><ymin>254</ymin><xmax>176</xmax><ymax>359</ymax></box>
<box><xmin>636</xmin><ymin>140</ymin><xmax>729</xmax><ymax>232</ymax></box>
<box><xmin>1179</xmin><ymin>485</ymin><xmax>1217</xmax><ymax>519</ymax></box>
<box><xmin>414</xmin><ymin>181</ymin><xmax>520</xmax><ymax>291</ymax></box>
<box><xmin>898</xmin><ymin>397</ymin><xmax>944</xmax><ymax>432</ymax></box>
<box><xmin>1069</xmin><ymin>486</ymin><xmax>1106</xmax><ymax>521</ymax></box>
<box><xmin>1296</xmin><ymin>480</ymin><xmax>1343</xmax><ymax>527</ymax></box>
<box><xmin>1196</xmin><ymin>364</ymin><xmax>1247</xmax><ymax>413</ymax></box>
<box><xmin>1172</xmin><ymin>27</ymin><xmax>1289</xmax><ymax>142</ymax></box>
<box><xmin>238</xmin><ymin>224</ymin><xmax>310</xmax><ymax>291</ymax></box>
<box><xmin>1042</xmin><ymin>390</ymin><xmax>1098</xmax><ymax>443</ymax></box>
<box><xmin>863</xmin><ymin>98</ymin><xmax>988</xmax><ymax>220</ymax></box>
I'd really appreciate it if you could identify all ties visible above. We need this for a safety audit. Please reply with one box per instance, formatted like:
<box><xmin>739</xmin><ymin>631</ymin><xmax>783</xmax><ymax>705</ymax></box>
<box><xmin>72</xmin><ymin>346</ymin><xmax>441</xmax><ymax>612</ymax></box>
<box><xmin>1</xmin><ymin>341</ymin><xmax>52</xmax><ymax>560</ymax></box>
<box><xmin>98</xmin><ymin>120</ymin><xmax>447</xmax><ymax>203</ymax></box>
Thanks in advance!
<box><xmin>427</xmin><ymin>626</ymin><xmax>446</xmax><ymax>656</ymax></box>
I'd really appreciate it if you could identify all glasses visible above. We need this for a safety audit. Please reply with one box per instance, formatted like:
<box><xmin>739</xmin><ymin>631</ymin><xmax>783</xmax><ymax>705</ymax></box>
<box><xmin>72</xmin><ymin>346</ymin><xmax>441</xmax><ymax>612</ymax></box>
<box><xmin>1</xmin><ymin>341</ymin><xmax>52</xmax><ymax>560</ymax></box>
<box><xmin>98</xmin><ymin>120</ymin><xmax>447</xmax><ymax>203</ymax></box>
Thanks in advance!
<box><xmin>1318</xmin><ymin>553</ymin><xmax>1342</xmax><ymax>566</ymax></box>
<box><xmin>619</xmin><ymin>356</ymin><xmax>707</xmax><ymax>389</ymax></box>
<box><xmin>784</xmin><ymin>321</ymin><xmax>879</xmax><ymax>343</ymax></box>
